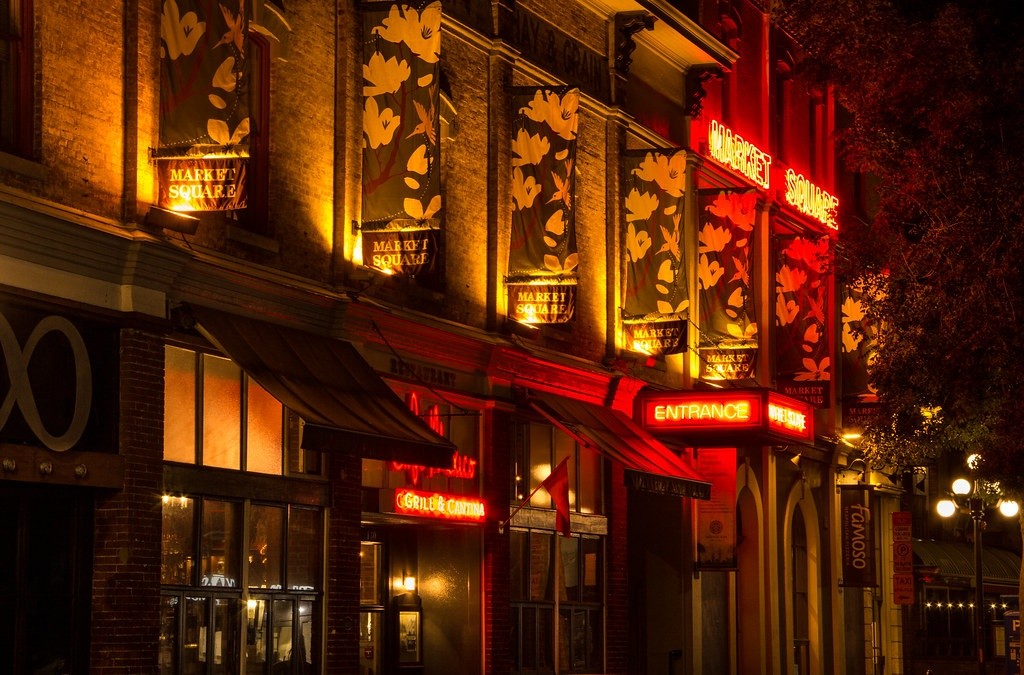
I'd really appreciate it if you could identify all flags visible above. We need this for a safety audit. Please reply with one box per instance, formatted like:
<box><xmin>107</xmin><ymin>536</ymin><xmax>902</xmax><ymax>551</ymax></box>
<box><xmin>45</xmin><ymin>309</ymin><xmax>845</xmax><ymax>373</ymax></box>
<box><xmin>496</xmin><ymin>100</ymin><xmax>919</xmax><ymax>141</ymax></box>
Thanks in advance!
<box><xmin>542</xmin><ymin>459</ymin><xmax>573</xmax><ymax>538</ymax></box>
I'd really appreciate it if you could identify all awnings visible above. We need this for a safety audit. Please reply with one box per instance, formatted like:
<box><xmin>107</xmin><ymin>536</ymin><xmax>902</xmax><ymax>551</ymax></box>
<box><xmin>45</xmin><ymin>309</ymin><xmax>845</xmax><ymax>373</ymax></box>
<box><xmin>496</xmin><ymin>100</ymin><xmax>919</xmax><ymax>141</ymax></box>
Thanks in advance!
<box><xmin>528</xmin><ymin>392</ymin><xmax>712</xmax><ymax>497</ymax></box>
<box><xmin>186</xmin><ymin>303</ymin><xmax>458</xmax><ymax>472</ymax></box>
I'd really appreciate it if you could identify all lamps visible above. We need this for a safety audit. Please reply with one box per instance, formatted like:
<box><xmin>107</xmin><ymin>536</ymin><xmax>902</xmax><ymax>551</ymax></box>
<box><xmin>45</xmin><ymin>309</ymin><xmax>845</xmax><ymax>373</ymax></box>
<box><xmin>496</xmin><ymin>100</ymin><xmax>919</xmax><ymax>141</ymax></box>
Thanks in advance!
<box><xmin>508</xmin><ymin>316</ymin><xmax>539</xmax><ymax>341</ymax></box>
<box><xmin>775</xmin><ymin>441</ymin><xmax>805</xmax><ymax>469</ymax></box>
<box><xmin>847</xmin><ymin>459</ymin><xmax>868</xmax><ymax>483</ymax></box>
<box><xmin>913</xmin><ymin>468</ymin><xmax>927</xmax><ymax>493</ymax></box>
<box><xmin>146</xmin><ymin>205</ymin><xmax>200</xmax><ymax>237</ymax></box>
<box><xmin>886</xmin><ymin>463</ymin><xmax>900</xmax><ymax>486</ymax></box>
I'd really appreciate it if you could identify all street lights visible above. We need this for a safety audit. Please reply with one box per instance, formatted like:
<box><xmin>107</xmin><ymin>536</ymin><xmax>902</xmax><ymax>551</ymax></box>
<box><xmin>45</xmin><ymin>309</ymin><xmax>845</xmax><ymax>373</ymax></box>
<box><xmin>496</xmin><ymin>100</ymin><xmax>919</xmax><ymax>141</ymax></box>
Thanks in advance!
<box><xmin>936</xmin><ymin>453</ymin><xmax>1020</xmax><ymax>675</ymax></box>
